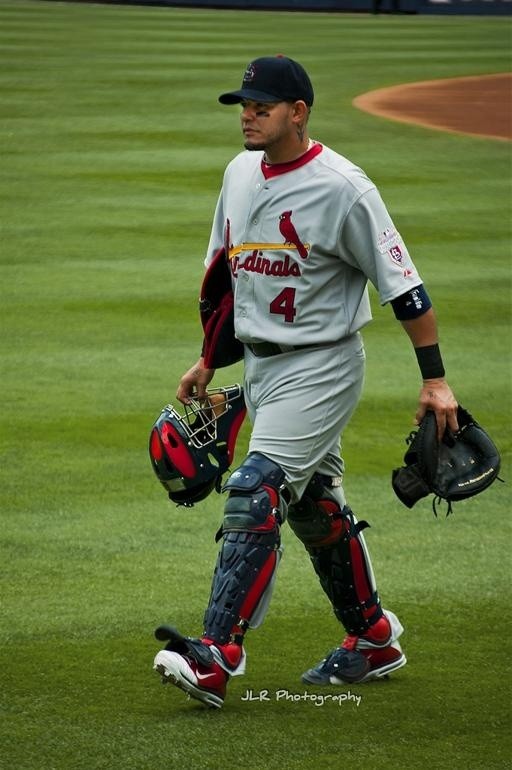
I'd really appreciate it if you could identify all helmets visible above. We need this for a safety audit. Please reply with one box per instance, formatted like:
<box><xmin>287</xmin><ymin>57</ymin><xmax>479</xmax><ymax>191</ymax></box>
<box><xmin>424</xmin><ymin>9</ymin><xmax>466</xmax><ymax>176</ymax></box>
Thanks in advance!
<box><xmin>149</xmin><ymin>383</ymin><xmax>246</xmax><ymax>508</ymax></box>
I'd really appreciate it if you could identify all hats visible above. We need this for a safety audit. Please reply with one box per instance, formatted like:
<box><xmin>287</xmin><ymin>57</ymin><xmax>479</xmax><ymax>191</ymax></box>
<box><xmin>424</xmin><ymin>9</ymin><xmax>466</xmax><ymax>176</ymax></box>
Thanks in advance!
<box><xmin>219</xmin><ymin>54</ymin><xmax>314</xmax><ymax>106</ymax></box>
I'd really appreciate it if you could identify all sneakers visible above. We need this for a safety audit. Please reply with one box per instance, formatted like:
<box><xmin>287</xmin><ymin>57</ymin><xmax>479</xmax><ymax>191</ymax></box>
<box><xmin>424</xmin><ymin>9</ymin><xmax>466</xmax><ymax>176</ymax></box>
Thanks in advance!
<box><xmin>152</xmin><ymin>650</ymin><xmax>229</xmax><ymax>709</ymax></box>
<box><xmin>301</xmin><ymin>636</ymin><xmax>407</xmax><ymax>686</ymax></box>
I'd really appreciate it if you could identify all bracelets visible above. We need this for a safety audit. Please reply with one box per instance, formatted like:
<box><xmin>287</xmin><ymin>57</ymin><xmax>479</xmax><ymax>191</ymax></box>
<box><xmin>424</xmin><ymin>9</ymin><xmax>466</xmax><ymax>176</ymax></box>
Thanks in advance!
<box><xmin>416</xmin><ymin>342</ymin><xmax>445</xmax><ymax>380</ymax></box>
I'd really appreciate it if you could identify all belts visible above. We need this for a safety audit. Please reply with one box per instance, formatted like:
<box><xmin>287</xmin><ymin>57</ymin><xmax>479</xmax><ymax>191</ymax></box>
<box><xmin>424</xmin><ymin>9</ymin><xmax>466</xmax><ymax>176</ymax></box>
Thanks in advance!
<box><xmin>246</xmin><ymin>341</ymin><xmax>312</xmax><ymax>358</ymax></box>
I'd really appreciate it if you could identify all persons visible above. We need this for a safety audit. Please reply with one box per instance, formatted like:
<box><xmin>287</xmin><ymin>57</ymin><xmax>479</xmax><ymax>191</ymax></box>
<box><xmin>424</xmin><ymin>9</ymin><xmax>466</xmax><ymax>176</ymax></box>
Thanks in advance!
<box><xmin>150</xmin><ymin>52</ymin><xmax>462</xmax><ymax>711</ymax></box>
